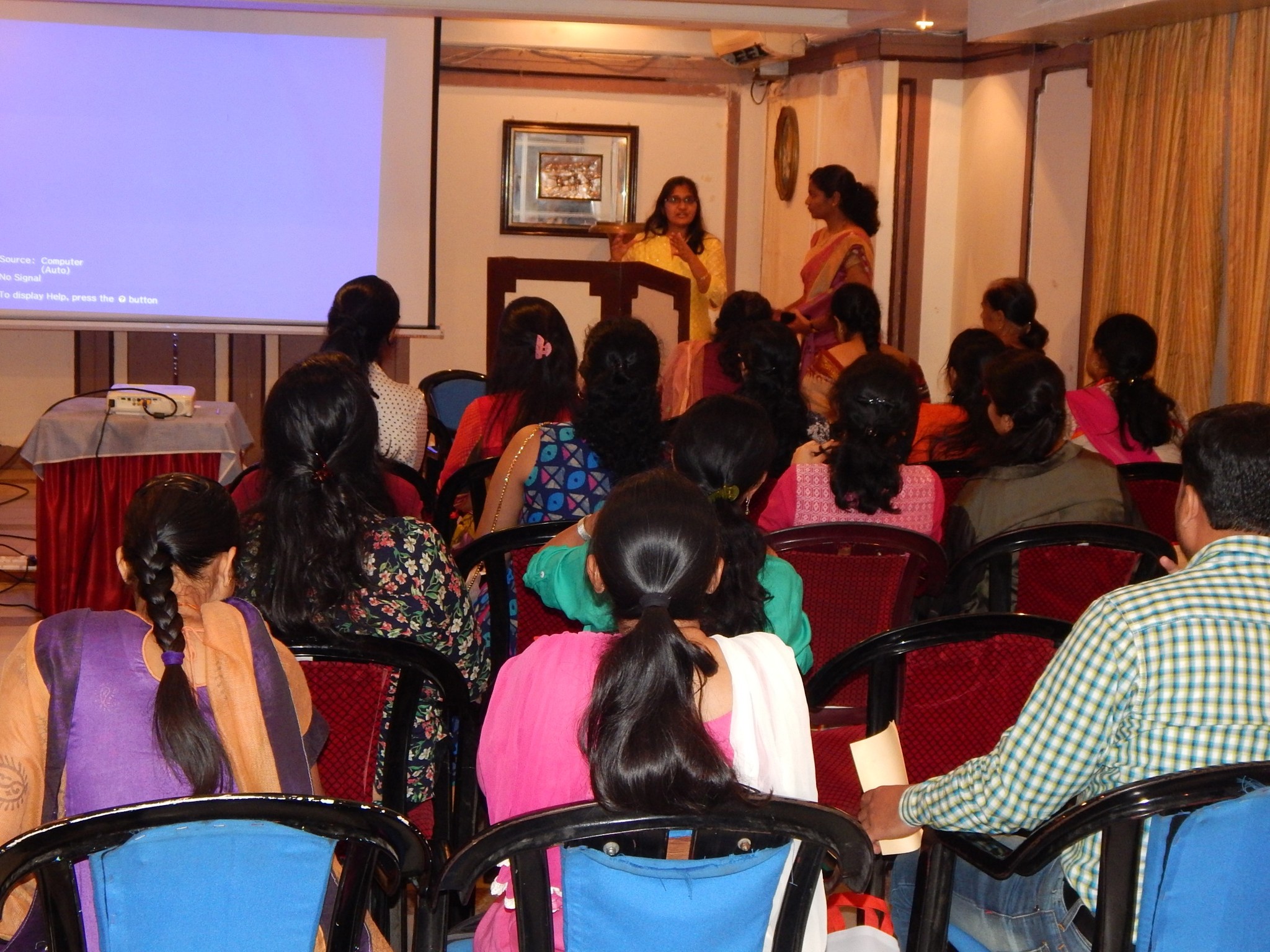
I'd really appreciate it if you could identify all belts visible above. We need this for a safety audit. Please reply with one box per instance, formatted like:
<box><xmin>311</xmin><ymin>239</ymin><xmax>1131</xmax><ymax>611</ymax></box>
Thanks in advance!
<box><xmin>1063</xmin><ymin>877</ymin><xmax>1136</xmax><ymax>952</ymax></box>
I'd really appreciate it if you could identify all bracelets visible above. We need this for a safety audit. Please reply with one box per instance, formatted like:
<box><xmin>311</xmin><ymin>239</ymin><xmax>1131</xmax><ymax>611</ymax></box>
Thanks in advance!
<box><xmin>576</xmin><ymin>514</ymin><xmax>593</xmax><ymax>541</ymax></box>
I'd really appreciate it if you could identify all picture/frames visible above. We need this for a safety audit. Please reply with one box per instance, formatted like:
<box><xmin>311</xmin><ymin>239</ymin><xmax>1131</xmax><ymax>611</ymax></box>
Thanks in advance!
<box><xmin>500</xmin><ymin>119</ymin><xmax>639</xmax><ymax>238</ymax></box>
<box><xmin>774</xmin><ymin>105</ymin><xmax>800</xmax><ymax>201</ymax></box>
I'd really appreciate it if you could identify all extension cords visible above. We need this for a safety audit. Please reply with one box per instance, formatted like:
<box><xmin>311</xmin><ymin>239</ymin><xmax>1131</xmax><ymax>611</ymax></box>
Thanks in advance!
<box><xmin>0</xmin><ymin>555</ymin><xmax>37</xmax><ymax>572</ymax></box>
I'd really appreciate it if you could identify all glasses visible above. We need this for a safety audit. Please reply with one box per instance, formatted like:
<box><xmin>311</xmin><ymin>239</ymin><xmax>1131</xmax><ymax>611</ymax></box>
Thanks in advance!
<box><xmin>668</xmin><ymin>195</ymin><xmax>694</xmax><ymax>204</ymax></box>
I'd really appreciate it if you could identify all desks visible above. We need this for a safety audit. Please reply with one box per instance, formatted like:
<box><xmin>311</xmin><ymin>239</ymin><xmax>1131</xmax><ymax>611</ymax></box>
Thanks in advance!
<box><xmin>20</xmin><ymin>397</ymin><xmax>254</xmax><ymax>617</ymax></box>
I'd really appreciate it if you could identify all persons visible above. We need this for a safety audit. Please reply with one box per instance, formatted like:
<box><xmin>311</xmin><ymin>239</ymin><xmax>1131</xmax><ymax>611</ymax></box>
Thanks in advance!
<box><xmin>473</xmin><ymin>473</ymin><xmax>827</xmax><ymax>952</ymax></box>
<box><xmin>769</xmin><ymin>164</ymin><xmax>881</xmax><ymax>427</ymax></box>
<box><xmin>312</xmin><ymin>272</ymin><xmax>1190</xmax><ymax>546</ymax></box>
<box><xmin>611</xmin><ymin>174</ymin><xmax>725</xmax><ymax>341</ymax></box>
<box><xmin>0</xmin><ymin>474</ymin><xmax>395</xmax><ymax>952</ymax></box>
<box><xmin>859</xmin><ymin>403</ymin><xmax>1270</xmax><ymax>952</ymax></box>
<box><xmin>227</xmin><ymin>364</ymin><xmax>818</xmax><ymax>817</ymax></box>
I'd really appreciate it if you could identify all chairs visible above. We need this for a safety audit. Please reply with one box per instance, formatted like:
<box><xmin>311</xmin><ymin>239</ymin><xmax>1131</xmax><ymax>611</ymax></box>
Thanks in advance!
<box><xmin>0</xmin><ymin>370</ymin><xmax>1270</xmax><ymax>952</ymax></box>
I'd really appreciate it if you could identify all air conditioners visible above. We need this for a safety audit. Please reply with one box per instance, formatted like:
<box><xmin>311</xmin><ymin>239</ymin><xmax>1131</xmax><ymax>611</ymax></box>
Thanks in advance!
<box><xmin>711</xmin><ymin>30</ymin><xmax>806</xmax><ymax>69</ymax></box>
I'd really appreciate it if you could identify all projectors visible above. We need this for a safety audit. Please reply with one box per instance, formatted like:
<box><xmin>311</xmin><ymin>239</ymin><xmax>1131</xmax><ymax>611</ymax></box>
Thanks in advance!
<box><xmin>110</xmin><ymin>384</ymin><xmax>196</xmax><ymax>417</ymax></box>
